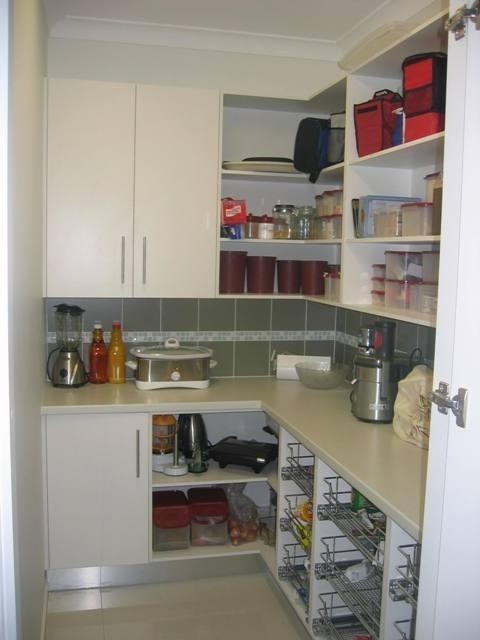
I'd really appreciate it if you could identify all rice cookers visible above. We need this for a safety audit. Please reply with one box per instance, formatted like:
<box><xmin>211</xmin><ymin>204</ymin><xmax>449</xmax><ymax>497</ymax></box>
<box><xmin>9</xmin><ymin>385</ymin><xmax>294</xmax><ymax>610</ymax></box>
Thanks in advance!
<box><xmin>125</xmin><ymin>336</ymin><xmax>219</xmax><ymax>391</ymax></box>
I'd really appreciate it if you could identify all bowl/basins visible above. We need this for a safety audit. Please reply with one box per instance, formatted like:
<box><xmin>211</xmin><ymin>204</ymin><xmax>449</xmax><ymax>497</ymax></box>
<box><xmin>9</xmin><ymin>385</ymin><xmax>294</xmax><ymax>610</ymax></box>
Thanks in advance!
<box><xmin>294</xmin><ymin>360</ymin><xmax>350</xmax><ymax>390</ymax></box>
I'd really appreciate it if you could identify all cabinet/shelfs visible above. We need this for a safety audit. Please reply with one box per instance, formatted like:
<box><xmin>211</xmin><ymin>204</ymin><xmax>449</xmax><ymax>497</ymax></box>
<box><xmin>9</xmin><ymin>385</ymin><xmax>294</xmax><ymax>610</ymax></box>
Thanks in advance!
<box><xmin>151</xmin><ymin>400</ymin><xmax>280</xmax><ymax>570</ymax></box>
<box><xmin>346</xmin><ymin>8</ymin><xmax>449</xmax><ymax>327</ymax></box>
<box><xmin>308</xmin><ymin>455</ymin><xmax>390</xmax><ymax>639</ymax></box>
<box><xmin>380</xmin><ymin>511</ymin><xmax>423</xmax><ymax>639</ymax></box>
<box><xmin>41</xmin><ymin>405</ymin><xmax>152</xmax><ymax>571</ymax></box>
<box><xmin>218</xmin><ymin>75</ymin><xmax>346</xmax><ymax>304</ymax></box>
<box><xmin>45</xmin><ymin>78</ymin><xmax>223</xmax><ymax>298</ymax></box>
<box><xmin>277</xmin><ymin>427</ymin><xmax>317</xmax><ymax>640</ymax></box>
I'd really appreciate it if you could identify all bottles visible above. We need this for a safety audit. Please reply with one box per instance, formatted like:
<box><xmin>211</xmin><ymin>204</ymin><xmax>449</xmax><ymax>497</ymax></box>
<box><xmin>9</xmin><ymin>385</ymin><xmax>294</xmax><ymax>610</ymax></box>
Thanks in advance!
<box><xmin>273</xmin><ymin>204</ymin><xmax>315</xmax><ymax>240</ymax></box>
<box><xmin>89</xmin><ymin>323</ymin><xmax>108</xmax><ymax>385</ymax></box>
<box><xmin>106</xmin><ymin>321</ymin><xmax>126</xmax><ymax>384</ymax></box>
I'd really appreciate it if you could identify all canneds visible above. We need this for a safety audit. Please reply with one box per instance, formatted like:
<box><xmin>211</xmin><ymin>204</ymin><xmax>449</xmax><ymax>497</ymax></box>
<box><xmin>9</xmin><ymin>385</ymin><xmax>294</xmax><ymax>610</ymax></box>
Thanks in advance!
<box><xmin>272</xmin><ymin>204</ymin><xmax>297</xmax><ymax>240</ymax></box>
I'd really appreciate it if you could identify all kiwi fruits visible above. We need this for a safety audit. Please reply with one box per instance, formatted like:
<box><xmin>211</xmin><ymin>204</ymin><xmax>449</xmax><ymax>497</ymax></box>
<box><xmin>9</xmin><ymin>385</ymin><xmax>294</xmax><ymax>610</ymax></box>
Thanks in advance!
<box><xmin>229</xmin><ymin>521</ymin><xmax>259</xmax><ymax>546</ymax></box>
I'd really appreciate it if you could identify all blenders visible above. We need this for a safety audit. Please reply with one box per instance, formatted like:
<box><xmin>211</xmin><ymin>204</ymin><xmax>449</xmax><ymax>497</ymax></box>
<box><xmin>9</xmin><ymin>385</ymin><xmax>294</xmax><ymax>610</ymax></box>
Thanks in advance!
<box><xmin>46</xmin><ymin>303</ymin><xmax>90</xmax><ymax>388</ymax></box>
<box><xmin>349</xmin><ymin>320</ymin><xmax>408</xmax><ymax>424</ymax></box>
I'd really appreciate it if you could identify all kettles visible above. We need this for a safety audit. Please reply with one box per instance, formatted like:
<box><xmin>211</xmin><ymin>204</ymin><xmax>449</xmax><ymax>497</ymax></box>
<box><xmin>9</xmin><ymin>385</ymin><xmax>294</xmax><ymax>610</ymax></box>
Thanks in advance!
<box><xmin>179</xmin><ymin>413</ymin><xmax>214</xmax><ymax>459</ymax></box>
